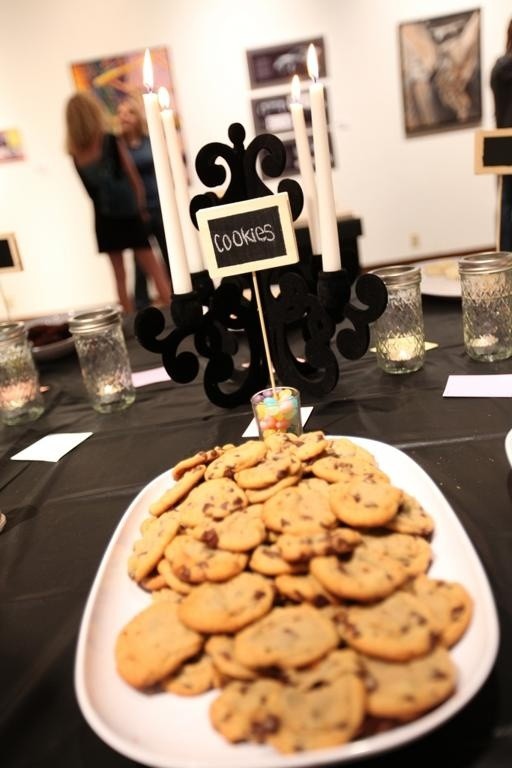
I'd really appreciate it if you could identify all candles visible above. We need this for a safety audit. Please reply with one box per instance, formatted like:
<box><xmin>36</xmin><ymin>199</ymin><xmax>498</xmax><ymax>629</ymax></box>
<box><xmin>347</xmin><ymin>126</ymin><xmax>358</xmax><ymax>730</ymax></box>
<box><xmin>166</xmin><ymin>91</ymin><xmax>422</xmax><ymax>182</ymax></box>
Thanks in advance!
<box><xmin>288</xmin><ymin>40</ymin><xmax>342</xmax><ymax>274</ymax></box>
<box><xmin>141</xmin><ymin>47</ymin><xmax>204</xmax><ymax>293</ymax></box>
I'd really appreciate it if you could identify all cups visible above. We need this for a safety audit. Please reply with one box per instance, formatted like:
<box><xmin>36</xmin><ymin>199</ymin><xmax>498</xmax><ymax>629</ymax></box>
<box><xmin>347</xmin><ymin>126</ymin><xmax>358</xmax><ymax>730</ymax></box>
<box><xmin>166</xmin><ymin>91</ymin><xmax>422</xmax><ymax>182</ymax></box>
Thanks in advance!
<box><xmin>0</xmin><ymin>320</ymin><xmax>43</xmax><ymax>427</ymax></box>
<box><xmin>367</xmin><ymin>263</ymin><xmax>429</xmax><ymax>376</ymax></box>
<box><xmin>65</xmin><ymin>308</ymin><xmax>136</xmax><ymax>415</ymax></box>
<box><xmin>455</xmin><ymin>250</ymin><xmax>512</xmax><ymax>365</ymax></box>
<box><xmin>251</xmin><ymin>385</ymin><xmax>304</xmax><ymax>443</ymax></box>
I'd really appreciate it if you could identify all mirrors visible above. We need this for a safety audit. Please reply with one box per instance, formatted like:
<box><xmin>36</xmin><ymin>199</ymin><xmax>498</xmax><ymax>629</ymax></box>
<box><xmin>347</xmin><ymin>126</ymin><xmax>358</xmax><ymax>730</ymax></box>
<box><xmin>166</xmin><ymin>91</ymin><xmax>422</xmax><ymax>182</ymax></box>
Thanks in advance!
<box><xmin>68</xmin><ymin>42</ymin><xmax>181</xmax><ymax>309</ymax></box>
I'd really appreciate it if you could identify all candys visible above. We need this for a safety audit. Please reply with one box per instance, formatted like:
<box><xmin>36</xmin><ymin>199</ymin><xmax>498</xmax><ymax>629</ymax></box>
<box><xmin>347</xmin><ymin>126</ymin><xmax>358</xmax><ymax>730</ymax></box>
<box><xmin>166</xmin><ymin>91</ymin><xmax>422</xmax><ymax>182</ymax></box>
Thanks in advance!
<box><xmin>253</xmin><ymin>387</ymin><xmax>299</xmax><ymax>440</ymax></box>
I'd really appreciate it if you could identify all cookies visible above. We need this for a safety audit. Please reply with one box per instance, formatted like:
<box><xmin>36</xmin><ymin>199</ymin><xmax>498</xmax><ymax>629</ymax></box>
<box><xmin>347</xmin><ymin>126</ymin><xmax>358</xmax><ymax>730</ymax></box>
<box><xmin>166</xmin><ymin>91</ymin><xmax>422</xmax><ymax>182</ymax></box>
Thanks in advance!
<box><xmin>116</xmin><ymin>429</ymin><xmax>473</xmax><ymax>754</ymax></box>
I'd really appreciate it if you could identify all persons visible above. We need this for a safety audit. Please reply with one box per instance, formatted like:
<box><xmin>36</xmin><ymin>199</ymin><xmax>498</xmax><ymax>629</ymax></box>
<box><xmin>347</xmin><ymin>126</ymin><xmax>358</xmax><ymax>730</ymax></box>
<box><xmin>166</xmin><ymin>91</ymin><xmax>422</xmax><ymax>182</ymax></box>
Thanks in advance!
<box><xmin>112</xmin><ymin>97</ymin><xmax>171</xmax><ymax>307</ymax></box>
<box><xmin>65</xmin><ymin>95</ymin><xmax>174</xmax><ymax>336</ymax></box>
<box><xmin>488</xmin><ymin>16</ymin><xmax>512</xmax><ymax>253</ymax></box>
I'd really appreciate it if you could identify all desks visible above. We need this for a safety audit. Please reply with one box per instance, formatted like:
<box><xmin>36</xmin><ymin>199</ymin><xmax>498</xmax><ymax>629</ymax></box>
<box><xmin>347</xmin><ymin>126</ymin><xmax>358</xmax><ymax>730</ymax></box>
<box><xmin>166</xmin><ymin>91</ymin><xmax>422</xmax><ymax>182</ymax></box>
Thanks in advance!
<box><xmin>3</xmin><ymin>314</ymin><xmax>511</xmax><ymax>764</ymax></box>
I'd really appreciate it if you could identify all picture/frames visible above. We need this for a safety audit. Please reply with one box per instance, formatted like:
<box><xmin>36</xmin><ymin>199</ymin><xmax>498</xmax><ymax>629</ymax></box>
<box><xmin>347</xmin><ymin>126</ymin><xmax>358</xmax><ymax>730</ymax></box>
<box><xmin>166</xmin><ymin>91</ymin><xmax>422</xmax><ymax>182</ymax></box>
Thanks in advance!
<box><xmin>396</xmin><ymin>5</ymin><xmax>488</xmax><ymax>140</ymax></box>
<box><xmin>242</xmin><ymin>34</ymin><xmax>337</xmax><ymax>176</ymax></box>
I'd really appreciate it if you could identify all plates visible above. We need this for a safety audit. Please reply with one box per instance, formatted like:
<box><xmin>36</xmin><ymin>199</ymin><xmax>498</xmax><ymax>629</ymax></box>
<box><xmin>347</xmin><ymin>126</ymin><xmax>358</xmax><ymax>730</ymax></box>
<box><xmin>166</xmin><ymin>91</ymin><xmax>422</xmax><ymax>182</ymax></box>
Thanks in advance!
<box><xmin>73</xmin><ymin>430</ymin><xmax>500</xmax><ymax>768</ymax></box>
